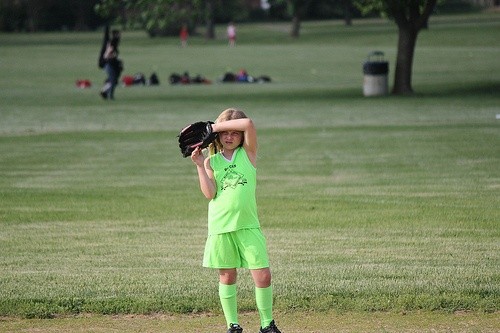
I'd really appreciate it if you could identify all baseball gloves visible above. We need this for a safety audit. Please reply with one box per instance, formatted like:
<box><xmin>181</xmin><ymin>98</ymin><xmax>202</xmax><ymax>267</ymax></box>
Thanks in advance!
<box><xmin>176</xmin><ymin>121</ymin><xmax>218</xmax><ymax>159</ymax></box>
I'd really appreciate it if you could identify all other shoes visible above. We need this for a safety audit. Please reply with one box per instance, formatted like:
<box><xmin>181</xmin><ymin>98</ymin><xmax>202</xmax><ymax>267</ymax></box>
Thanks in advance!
<box><xmin>227</xmin><ymin>323</ymin><xmax>243</xmax><ymax>333</ymax></box>
<box><xmin>258</xmin><ymin>320</ymin><xmax>281</xmax><ymax>333</ymax></box>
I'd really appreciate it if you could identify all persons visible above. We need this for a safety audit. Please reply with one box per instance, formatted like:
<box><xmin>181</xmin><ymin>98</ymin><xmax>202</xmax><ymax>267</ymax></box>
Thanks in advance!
<box><xmin>100</xmin><ymin>22</ymin><xmax>236</xmax><ymax>99</ymax></box>
<box><xmin>177</xmin><ymin>108</ymin><xmax>282</xmax><ymax>333</ymax></box>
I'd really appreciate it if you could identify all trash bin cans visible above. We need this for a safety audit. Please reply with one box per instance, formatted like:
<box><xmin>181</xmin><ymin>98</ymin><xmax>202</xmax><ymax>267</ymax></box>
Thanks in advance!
<box><xmin>362</xmin><ymin>51</ymin><xmax>389</xmax><ymax>97</ymax></box>
